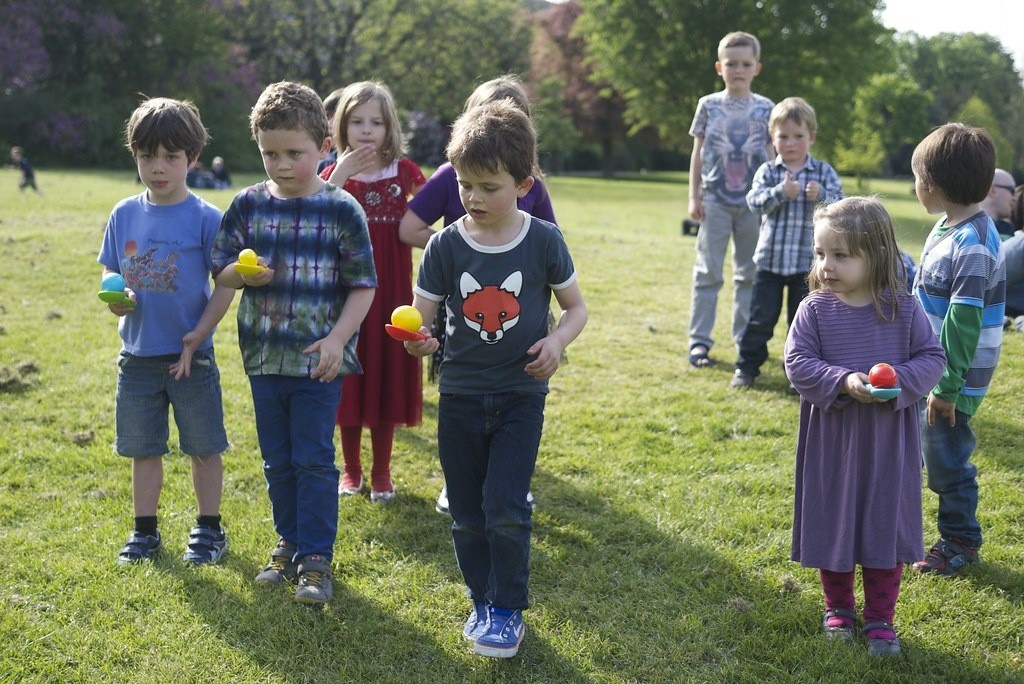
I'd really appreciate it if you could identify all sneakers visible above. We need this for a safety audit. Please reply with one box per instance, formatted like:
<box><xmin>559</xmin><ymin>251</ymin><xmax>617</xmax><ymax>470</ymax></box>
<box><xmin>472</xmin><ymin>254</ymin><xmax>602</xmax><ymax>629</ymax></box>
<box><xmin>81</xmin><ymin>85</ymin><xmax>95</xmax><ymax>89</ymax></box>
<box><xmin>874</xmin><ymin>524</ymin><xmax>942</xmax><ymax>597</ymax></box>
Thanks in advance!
<box><xmin>117</xmin><ymin>530</ymin><xmax>161</xmax><ymax>567</ymax></box>
<box><xmin>183</xmin><ymin>525</ymin><xmax>227</xmax><ymax>566</ymax></box>
<box><xmin>913</xmin><ymin>537</ymin><xmax>980</xmax><ymax>576</ymax></box>
<box><xmin>462</xmin><ymin>602</ymin><xmax>525</xmax><ymax>658</ymax></box>
<box><xmin>788</xmin><ymin>382</ymin><xmax>799</xmax><ymax>395</ymax></box>
<box><xmin>254</xmin><ymin>539</ymin><xmax>297</xmax><ymax>586</ymax></box>
<box><xmin>731</xmin><ymin>369</ymin><xmax>754</xmax><ymax>390</ymax></box>
<box><xmin>435</xmin><ymin>481</ymin><xmax>452</xmax><ymax>515</ymax></box>
<box><xmin>295</xmin><ymin>554</ymin><xmax>333</xmax><ymax>603</ymax></box>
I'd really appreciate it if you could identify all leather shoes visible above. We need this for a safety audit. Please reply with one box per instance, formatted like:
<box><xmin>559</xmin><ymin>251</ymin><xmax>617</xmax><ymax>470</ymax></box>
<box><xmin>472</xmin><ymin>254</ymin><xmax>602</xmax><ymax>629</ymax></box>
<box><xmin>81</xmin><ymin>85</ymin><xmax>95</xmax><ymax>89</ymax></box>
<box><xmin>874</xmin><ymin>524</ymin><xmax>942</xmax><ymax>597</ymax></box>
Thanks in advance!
<box><xmin>861</xmin><ymin>621</ymin><xmax>901</xmax><ymax>658</ymax></box>
<box><xmin>370</xmin><ymin>480</ymin><xmax>394</xmax><ymax>506</ymax></box>
<box><xmin>337</xmin><ymin>475</ymin><xmax>363</xmax><ymax>496</ymax></box>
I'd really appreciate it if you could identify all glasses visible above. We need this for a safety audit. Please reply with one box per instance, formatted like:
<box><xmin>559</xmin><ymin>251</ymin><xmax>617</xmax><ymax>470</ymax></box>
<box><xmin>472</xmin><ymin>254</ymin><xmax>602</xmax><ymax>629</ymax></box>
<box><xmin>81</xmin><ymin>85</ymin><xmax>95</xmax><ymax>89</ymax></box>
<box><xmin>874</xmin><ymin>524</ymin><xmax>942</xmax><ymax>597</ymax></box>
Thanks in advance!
<box><xmin>994</xmin><ymin>184</ymin><xmax>1015</xmax><ymax>196</ymax></box>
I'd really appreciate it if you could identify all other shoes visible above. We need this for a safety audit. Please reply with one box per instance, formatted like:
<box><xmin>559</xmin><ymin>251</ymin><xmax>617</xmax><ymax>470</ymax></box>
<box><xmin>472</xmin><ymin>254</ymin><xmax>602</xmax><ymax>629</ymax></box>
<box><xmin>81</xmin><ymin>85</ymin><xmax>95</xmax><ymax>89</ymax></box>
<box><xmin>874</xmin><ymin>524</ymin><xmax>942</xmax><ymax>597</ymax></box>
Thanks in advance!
<box><xmin>824</xmin><ymin>610</ymin><xmax>858</xmax><ymax>645</ymax></box>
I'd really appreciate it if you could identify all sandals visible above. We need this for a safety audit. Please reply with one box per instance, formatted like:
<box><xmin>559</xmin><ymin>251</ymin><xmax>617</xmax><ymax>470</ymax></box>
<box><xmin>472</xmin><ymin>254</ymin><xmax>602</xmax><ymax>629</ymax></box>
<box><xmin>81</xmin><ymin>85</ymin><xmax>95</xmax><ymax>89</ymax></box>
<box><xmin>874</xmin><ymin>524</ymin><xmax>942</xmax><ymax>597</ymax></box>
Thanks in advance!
<box><xmin>688</xmin><ymin>343</ymin><xmax>714</xmax><ymax>369</ymax></box>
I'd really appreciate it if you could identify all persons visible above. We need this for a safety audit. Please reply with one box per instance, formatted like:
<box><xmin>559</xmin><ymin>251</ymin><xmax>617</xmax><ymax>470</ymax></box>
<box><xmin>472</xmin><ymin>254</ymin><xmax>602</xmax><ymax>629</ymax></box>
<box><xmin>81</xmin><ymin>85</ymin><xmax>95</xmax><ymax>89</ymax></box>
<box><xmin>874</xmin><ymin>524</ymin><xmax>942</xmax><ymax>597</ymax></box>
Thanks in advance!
<box><xmin>321</xmin><ymin>80</ymin><xmax>425</xmax><ymax>498</ymax></box>
<box><xmin>385</xmin><ymin>98</ymin><xmax>588</xmax><ymax>658</ymax></box>
<box><xmin>912</xmin><ymin>123</ymin><xmax>1007</xmax><ymax>571</ymax></box>
<box><xmin>189</xmin><ymin>158</ymin><xmax>231</xmax><ymax>189</ymax></box>
<box><xmin>98</xmin><ymin>98</ymin><xmax>235</xmax><ymax>566</ymax></box>
<box><xmin>211</xmin><ymin>82</ymin><xmax>377</xmax><ymax>603</ymax></box>
<box><xmin>730</xmin><ymin>98</ymin><xmax>844</xmax><ymax>393</ymax></box>
<box><xmin>785</xmin><ymin>196</ymin><xmax>947</xmax><ymax>660</ymax></box>
<box><xmin>984</xmin><ymin>169</ymin><xmax>1024</xmax><ymax>315</ymax></box>
<box><xmin>9</xmin><ymin>146</ymin><xmax>41</xmax><ymax>195</ymax></box>
<box><xmin>686</xmin><ymin>31</ymin><xmax>775</xmax><ymax>367</ymax></box>
<box><xmin>401</xmin><ymin>75</ymin><xmax>557</xmax><ymax>517</ymax></box>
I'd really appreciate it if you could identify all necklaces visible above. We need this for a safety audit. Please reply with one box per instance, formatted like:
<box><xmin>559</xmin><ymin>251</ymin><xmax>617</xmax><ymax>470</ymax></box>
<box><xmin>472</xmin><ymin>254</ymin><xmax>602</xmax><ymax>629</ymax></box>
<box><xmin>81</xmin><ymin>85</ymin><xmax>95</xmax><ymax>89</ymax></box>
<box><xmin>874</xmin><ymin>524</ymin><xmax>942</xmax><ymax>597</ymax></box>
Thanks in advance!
<box><xmin>359</xmin><ymin>165</ymin><xmax>383</xmax><ymax>194</ymax></box>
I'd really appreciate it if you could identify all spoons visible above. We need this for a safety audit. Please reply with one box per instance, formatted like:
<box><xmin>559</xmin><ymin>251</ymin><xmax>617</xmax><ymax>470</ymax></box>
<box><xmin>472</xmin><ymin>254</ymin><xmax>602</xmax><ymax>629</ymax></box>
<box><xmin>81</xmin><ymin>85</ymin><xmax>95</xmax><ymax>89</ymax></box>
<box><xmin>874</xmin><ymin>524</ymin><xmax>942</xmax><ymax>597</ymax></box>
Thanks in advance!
<box><xmin>233</xmin><ymin>263</ymin><xmax>269</xmax><ymax>277</ymax></box>
<box><xmin>863</xmin><ymin>383</ymin><xmax>901</xmax><ymax>400</ymax></box>
<box><xmin>384</xmin><ymin>323</ymin><xmax>427</xmax><ymax>342</ymax></box>
<box><xmin>98</xmin><ymin>290</ymin><xmax>138</xmax><ymax>308</ymax></box>
